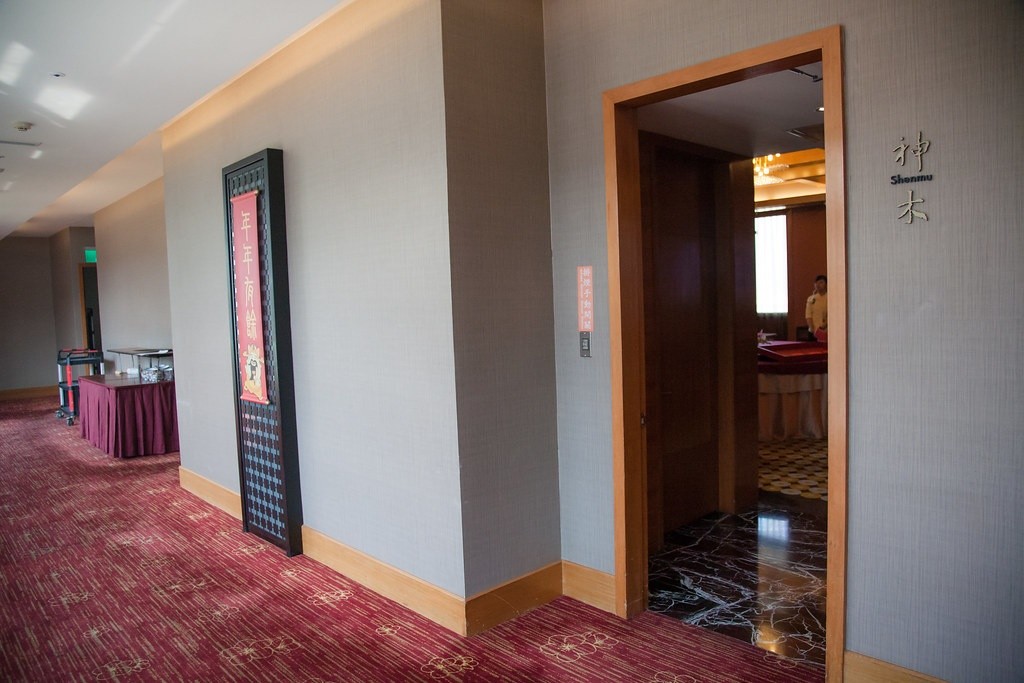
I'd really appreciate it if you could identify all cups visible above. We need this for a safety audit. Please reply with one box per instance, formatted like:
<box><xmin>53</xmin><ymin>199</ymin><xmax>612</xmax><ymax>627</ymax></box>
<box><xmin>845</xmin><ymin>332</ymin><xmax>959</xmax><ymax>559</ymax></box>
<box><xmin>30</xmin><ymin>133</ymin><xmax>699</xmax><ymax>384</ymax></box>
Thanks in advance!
<box><xmin>141</xmin><ymin>364</ymin><xmax>173</xmax><ymax>383</ymax></box>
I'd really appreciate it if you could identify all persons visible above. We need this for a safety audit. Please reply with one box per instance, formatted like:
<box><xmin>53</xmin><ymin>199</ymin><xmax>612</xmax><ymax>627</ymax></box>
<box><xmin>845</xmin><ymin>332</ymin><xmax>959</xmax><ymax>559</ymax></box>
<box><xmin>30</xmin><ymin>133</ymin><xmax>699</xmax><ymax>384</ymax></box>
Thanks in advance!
<box><xmin>806</xmin><ymin>275</ymin><xmax>827</xmax><ymax>342</ymax></box>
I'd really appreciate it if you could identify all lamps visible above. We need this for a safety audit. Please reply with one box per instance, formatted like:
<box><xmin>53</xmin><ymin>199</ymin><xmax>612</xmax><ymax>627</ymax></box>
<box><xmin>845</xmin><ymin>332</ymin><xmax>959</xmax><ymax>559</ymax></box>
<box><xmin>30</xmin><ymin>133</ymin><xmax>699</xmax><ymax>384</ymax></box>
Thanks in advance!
<box><xmin>754</xmin><ymin>153</ymin><xmax>789</xmax><ymax>187</ymax></box>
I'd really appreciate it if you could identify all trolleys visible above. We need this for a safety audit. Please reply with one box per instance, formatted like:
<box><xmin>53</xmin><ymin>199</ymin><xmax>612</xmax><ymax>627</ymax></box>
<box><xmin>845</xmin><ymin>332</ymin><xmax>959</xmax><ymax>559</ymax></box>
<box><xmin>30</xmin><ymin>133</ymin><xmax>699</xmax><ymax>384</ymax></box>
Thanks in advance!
<box><xmin>54</xmin><ymin>347</ymin><xmax>104</xmax><ymax>425</ymax></box>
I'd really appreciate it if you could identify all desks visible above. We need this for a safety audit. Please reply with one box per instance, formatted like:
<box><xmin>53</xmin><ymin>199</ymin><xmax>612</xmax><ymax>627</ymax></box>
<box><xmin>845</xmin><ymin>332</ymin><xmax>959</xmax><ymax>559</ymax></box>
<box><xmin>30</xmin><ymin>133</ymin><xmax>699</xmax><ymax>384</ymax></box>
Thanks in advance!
<box><xmin>758</xmin><ymin>339</ymin><xmax>828</xmax><ymax>444</ymax></box>
<box><xmin>79</xmin><ymin>372</ymin><xmax>178</xmax><ymax>461</ymax></box>
